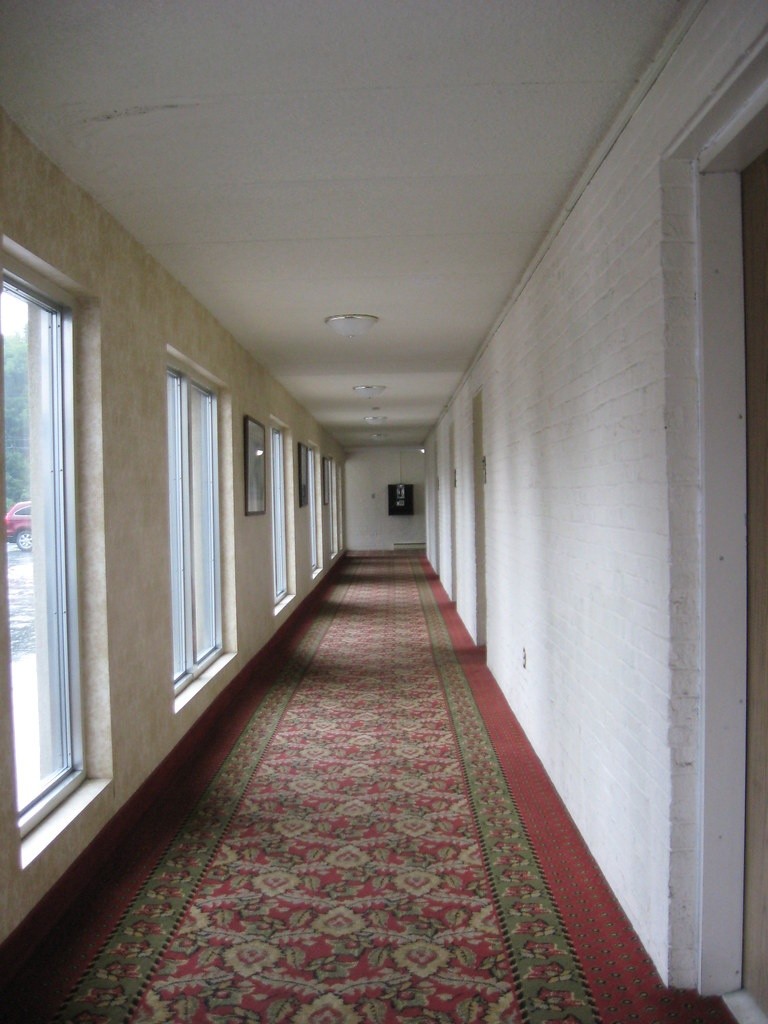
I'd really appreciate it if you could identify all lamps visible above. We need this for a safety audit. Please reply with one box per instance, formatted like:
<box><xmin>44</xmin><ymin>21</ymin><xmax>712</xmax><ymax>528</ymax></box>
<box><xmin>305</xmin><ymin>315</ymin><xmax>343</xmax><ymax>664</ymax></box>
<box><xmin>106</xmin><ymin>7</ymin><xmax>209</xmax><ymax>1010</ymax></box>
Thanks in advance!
<box><xmin>325</xmin><ymin>313</ymin><xmax>380</xmax><ymax>339</ymax></box>
<box><xmin>365</xmin><ymin>417</ymin><xmax>388</xmax><ymax>425</ymax></box>
<box><xmin>352</xmin><ymin>386</ymin><xmax>386</xmax><ymax>400</ymax></box>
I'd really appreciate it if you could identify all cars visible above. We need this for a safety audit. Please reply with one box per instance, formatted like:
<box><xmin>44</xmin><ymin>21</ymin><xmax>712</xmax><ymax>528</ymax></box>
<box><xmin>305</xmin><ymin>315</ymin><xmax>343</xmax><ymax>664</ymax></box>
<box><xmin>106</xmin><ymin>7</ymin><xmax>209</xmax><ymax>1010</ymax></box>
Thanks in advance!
<box><xmin>4</xmin><ymin>500</ymin><xmax>33</xmax><ymax>553</ymax></box>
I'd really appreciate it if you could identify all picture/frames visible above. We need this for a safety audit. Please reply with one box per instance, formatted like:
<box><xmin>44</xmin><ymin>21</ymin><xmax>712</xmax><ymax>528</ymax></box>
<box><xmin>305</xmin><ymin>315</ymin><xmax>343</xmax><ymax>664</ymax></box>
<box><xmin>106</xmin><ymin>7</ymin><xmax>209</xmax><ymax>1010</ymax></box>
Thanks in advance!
<box><xmin>298</xmin><ymin>442</ymin><xmax>308</xmax><ymax>508</ymax></box>
<box><xmin>244</xmin><ymin>414</ymin><xmax>266</xmax><ymax>516</ymax></box>
<box><xmin>324</xmin><ymin>457</ymin><xmax>329</xmax><ymax>505</ymax></box>
